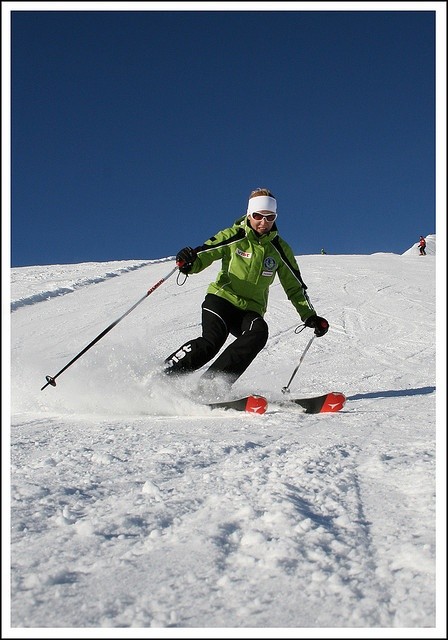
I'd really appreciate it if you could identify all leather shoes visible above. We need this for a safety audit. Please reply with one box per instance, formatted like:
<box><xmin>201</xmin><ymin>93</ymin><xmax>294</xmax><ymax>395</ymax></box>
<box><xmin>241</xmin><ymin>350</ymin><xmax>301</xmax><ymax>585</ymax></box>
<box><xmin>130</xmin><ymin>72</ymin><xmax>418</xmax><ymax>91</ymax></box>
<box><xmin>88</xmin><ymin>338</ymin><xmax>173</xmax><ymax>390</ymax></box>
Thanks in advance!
<box><xmin>295</xmin><ymin>315</ymin><xmax>329</xmax><ymax>337</ymax></box>
<box><xmin>177</xmin><ymin>247</ymin><xmax>197</xmax><ymax>287</ymax></box>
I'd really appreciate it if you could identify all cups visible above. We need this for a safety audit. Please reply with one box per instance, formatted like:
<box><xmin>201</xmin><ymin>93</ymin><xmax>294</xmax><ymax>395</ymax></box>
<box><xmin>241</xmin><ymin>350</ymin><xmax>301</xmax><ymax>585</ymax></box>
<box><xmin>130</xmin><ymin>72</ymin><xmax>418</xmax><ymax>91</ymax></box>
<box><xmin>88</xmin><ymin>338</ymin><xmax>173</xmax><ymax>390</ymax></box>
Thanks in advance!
<box><xmin>252</xmin><ymin>212</ymin><xmax>277</xmax><ymax>222</ymax></box>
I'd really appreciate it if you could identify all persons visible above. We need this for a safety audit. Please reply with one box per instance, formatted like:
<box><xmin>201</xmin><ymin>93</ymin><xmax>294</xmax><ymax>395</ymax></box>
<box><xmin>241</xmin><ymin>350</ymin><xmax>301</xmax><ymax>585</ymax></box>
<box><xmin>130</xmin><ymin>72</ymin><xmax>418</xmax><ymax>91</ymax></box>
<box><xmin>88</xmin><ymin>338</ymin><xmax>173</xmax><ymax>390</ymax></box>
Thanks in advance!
<box><xmin>162</xmin><ymin>188</ymin><xmax>328</xmax><ymax>399</ymax></box>
<box><xmin>417</xmin><ymin>235</ymin><xmax>425</xmax><ymax>255</ymax></box>
